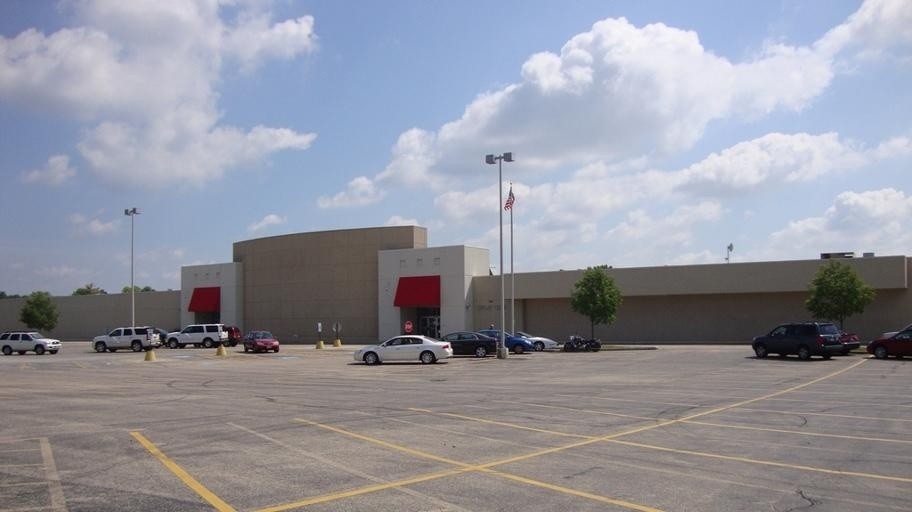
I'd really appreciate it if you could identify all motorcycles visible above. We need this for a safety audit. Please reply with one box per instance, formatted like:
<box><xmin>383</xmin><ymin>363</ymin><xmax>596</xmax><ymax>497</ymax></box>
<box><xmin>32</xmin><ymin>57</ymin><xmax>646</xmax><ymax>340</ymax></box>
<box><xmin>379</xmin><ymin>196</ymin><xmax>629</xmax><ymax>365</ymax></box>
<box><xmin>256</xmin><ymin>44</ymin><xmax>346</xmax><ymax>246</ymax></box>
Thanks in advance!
<box><xmin>564</xmin><ymin>333</ymin><xmax>602</xmax><ymax>353</ymax></box>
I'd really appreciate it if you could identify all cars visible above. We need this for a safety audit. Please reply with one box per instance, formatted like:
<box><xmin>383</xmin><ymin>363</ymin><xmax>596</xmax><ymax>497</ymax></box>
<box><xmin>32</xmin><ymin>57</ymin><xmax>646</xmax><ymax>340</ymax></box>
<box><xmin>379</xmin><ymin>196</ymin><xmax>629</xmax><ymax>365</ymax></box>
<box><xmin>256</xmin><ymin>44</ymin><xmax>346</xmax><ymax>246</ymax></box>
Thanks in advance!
<box><xmin>866</xmin><ymin>324</ymin><xmax>912</xmax><ymax>360</ymax></box>
<box><xmin>837</xmin><ymin>329</ymin><xmax>860</xmax><ymax>356</ymax></box>
<box><xmin>243</xmin><ymin>330</ymin><xmax>280</xmax><ymax>353</ymax></box>
<box><xmin>352</xmin><ymin>331</ymin><xmax>562</xmax><ymax>366</ymax></box>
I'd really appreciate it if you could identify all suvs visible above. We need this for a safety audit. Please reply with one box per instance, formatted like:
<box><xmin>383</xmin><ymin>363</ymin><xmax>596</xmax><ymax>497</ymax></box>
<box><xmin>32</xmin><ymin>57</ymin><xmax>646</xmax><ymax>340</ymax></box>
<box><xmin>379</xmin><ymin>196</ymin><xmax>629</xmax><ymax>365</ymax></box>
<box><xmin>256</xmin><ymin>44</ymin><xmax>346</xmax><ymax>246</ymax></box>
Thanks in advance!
<box><xmin>1</xmin><ymin>331</ymin><xmax>63</xmax><ymax>355</ymax></box>
<box><xmin>752</xmin><ymin>320</ymin><xmax>844</xmax><ymax>360</ymax></box>
<box><xmin>91</xmin><ymin>324</ymin><xmax>242</xmax><ymax>355</ymax></box>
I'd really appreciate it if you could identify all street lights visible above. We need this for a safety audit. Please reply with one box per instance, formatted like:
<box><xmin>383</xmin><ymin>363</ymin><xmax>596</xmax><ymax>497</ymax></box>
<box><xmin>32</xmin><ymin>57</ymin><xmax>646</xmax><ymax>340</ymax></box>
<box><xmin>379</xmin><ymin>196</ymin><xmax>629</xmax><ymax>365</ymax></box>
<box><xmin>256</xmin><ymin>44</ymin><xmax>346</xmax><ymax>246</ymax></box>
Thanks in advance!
<box><xmin>124</xmin><ymin>206</ymin><xmax>142</xmax><ymax>327</ymax></box>
<box><xmin>485</xmin><ymin>152</ymin><xmax>515</xmax><ymax>358</ymax></box>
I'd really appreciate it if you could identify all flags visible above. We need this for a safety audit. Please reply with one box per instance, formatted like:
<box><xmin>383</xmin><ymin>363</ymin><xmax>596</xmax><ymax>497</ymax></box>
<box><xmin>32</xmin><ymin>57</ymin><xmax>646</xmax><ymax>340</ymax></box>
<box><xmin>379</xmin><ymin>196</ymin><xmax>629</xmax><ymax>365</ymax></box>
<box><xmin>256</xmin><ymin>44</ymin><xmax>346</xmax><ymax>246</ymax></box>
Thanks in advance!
<box><xmin>503</xmin><ymin>191</ymin><xmax>515</xmax><ymax>211</ymax></box>
